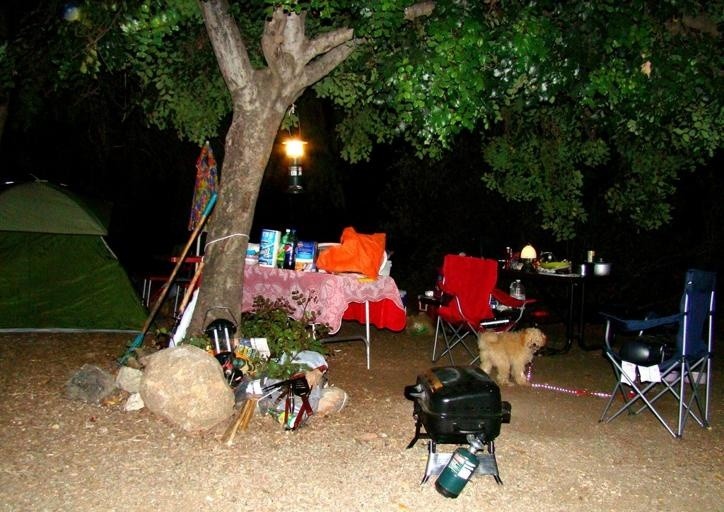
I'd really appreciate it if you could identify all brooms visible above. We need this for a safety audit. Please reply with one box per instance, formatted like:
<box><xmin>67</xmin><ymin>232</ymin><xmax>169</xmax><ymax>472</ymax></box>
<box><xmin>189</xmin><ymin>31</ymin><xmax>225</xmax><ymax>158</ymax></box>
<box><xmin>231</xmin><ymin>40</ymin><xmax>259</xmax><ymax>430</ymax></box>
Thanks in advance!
<box><xmin>119</xmin><ymin>193</ymin><xmax>218</xmax><ymax>363</ymax></box>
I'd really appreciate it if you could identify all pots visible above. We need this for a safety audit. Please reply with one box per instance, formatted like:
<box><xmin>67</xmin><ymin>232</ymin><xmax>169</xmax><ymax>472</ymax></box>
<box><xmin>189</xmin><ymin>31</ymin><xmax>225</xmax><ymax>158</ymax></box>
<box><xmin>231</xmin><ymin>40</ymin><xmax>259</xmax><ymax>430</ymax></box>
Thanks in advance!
<box><xmin>594</xmin><ymin>258</ymin><xmax>611</xmax><ymax>276</ymax></box>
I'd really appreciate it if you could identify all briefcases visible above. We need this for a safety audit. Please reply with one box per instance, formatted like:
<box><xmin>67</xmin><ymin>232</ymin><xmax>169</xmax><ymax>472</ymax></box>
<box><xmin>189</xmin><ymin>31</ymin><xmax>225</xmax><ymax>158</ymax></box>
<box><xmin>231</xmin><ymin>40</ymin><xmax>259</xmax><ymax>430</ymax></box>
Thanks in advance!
<box><xmin>619</xmin><ymin>334</ymin><xmax>678</xmax><ymax>368</ymax></box>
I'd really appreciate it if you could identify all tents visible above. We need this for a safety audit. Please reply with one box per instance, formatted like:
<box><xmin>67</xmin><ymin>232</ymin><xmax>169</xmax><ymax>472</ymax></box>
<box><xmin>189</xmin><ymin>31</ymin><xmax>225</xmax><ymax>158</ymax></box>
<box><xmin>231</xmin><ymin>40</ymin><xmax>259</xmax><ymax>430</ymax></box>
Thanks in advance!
<box><xmin>1</xmin><ymin>178</ymin><xmax>166</xmax><ymax>342</ymax></box>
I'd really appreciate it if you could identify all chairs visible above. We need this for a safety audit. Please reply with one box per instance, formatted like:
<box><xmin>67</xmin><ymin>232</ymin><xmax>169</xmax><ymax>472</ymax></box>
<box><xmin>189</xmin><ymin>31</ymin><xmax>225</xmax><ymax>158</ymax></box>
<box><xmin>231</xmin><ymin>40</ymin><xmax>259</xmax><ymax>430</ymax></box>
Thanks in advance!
<box><xmin>143</xmin><ymin>276</ymin><xmax>190</xmax><ymax>320</ymax></box>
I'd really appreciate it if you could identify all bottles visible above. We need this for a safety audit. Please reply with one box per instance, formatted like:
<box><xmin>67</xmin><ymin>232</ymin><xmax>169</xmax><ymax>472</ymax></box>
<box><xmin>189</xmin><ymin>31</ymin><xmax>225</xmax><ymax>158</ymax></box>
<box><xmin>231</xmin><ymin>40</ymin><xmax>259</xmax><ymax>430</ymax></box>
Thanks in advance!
<box><xmin>509</xmin><ymin>279</ymin><xmax>526</xmax><ymax>301</ymax></box>
<box><xmin>505</xmin><ymin>247</ymin><xmax>521</xmax><ymax>270</ymax></box>
<box><xmin>435</xmin><ymin>433</ymin><xmax>482</xmax><ymax>498</ymax></box>
<box><xmin>276</xmin><ymin>229</ymin><xmax>290</xmax><ymax>269</ymax></box>
<box><xmin>267</xmin><ymin>407</ymin><xmax>297</xmax><ymax>429</ymax></box>
<box><xmin>284</xmin><ymin>228</ymin><xmax>296</xmax><ymax>270</ymax></box>
<box><xmin>490</xmin><ymin>298</ymin><xmax>497</xmax><ymax>309</ymax></box>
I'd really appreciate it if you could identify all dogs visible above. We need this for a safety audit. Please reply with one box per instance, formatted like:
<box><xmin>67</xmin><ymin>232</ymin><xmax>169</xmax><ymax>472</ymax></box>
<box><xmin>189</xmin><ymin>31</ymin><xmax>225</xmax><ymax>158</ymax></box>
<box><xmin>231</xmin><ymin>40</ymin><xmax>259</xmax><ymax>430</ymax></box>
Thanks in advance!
<box><xmin>478</xmin><ymin>327</ymin><xmax>546</xmax><ymax>386</ymax></box>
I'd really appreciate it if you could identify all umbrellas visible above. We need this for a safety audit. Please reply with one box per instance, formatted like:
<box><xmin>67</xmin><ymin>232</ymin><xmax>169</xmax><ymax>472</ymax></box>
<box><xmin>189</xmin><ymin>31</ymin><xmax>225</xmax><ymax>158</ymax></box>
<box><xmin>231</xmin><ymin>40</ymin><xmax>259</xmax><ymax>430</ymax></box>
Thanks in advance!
<box><xmin>186</xmin><ymin>143</ymin><xmax>224</xmax><ymax>263</ymax></box>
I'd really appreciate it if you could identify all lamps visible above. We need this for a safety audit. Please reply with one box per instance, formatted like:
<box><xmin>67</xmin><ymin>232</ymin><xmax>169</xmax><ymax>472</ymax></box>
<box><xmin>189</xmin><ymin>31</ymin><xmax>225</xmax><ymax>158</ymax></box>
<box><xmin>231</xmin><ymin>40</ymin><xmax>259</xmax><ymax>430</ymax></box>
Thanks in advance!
<box><xmin>282</xmin><ymin>119</ymin><xmax>309</xmax><ymax>194</ymax></box>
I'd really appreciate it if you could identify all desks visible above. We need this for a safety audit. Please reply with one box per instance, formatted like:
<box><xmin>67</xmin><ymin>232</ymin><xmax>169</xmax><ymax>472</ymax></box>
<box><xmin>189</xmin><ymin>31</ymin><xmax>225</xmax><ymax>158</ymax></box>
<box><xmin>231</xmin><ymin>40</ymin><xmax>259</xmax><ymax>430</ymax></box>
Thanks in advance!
<box><xmin>244</xmin><ymin>265</ymin><xmax>408</xmax><ymax>370</ymax></box>
